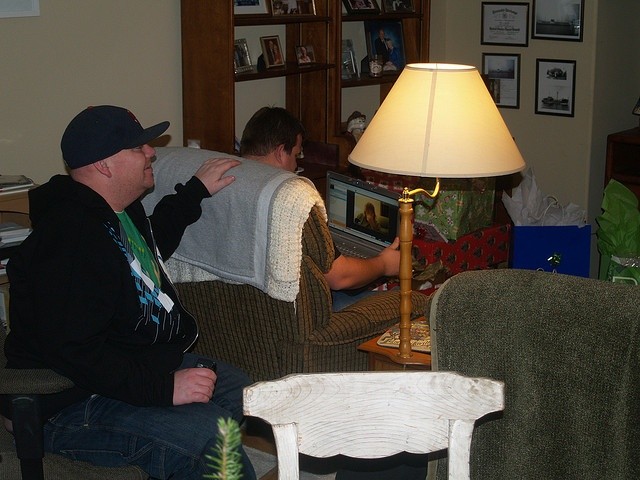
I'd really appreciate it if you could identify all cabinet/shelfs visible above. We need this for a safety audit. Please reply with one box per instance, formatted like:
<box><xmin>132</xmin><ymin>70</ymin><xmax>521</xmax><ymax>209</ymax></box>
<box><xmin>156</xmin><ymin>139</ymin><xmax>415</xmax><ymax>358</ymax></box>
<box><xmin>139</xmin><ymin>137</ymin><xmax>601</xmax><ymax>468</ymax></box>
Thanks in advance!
<box><xmin>336</xmin><ymin>0</ymin><xmax>431</xmax><ymax>180</ymax></box>
<box><xmin>180</xmin><ymin>0</ymin><xmax>346</xmax><ymax>206</ymax></box>
<box><xmin>604</xmin><ymin>125</ymin><xmax>640</xmax><ymax>203</ymax></box>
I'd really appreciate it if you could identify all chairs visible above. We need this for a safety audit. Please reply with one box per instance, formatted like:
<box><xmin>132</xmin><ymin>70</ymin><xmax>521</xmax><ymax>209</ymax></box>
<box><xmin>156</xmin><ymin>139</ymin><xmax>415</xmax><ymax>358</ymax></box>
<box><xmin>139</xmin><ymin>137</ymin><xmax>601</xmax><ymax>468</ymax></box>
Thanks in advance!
<box><xmin>0</xmin><ymin>282</ymin><xmax>148</xmax><ymax>480</ymax></box>
<box><xmin>243</xmin><ymin>371</ymin><xmax>505</xmax><ymax>479</ymax></box>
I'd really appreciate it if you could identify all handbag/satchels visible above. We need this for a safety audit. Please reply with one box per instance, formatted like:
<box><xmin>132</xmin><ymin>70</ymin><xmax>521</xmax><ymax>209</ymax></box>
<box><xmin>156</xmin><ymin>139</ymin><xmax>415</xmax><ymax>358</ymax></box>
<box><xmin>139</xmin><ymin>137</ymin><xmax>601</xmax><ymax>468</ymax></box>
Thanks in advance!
<box><xmin>511</xmin><ymin>196</ymin><xmax>591</xmax><ymax>279</ymax></box>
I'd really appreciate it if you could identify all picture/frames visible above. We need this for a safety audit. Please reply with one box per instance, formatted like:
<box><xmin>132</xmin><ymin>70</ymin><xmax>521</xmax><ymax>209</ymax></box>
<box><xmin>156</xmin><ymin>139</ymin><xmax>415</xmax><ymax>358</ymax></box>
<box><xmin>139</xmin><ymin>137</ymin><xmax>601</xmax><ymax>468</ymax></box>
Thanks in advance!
<box><xmin>235</xmin><ymin>0</ymin><xmax>267</xmax><ymax>14</ymax></box>
<box><xmin>481</xmin><ymin>2</ymin><xmax>530</xmax><ymax>47</ymax></box>
<box><xmin>294</xmin><ymin>44</ymin><xmax>317</xmax><ymax>66</ymax></box>
<box><xmin>382</xmin><ymin>1</ymin><xmax>414</xmax><ymax>13</ymax></box>
<box><xmin>234</xmin><ymin>38</ymin><xmax>254</xmax><ymax>74</ymax></box>
<box><xmin>343</xmin><ymin>0</ymin><xmax>380</xmax><ymax>14</ymax></box>
<box><xmin>535</xmin><ymin>58</ymin><xmax>576</xmax><ymax>118</ymax></box>
<box><xmin>532</xmin><ymin>0</ymin><xmax>586</xmax><ymax>42</ymax></box>
<box><xmin>271</xmin><ymin>0</ymin><xmax>302</xmax><ymax>16</ymax></box>
<box><xmin>482</xmin><ymin>52</ymin><xmax>520</xmax><ymax>108</ymax></box>
<box><xmin>260</xmin><ymin>35</ymin><xmax>286</xmax><ymax>68</ymax></box>
<box><xmin>366</xmin><ymin>19</ymin><xmax>405</xmax><ymax>76</ymax></box>
<box><xmin>342</xmin><ymin>50</ymin><xmax>357</xmax><ymax>80</ymax></box>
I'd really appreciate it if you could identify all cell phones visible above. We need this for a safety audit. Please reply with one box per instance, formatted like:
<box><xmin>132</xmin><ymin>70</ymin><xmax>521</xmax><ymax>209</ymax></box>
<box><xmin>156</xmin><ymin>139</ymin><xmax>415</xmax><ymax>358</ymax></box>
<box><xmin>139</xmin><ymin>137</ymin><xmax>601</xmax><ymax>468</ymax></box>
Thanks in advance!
<box><xmin>194</xmin><ymin>357</ymin><xmax>217</xmax><ymax>372</ymax></box>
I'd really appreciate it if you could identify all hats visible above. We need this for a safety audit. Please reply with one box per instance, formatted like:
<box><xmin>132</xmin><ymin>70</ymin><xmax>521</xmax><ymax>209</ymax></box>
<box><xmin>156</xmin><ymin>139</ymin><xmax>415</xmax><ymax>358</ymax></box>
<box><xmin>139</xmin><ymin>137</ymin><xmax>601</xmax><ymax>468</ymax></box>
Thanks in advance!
<box><xmin>61</xmin><ymin>106</ymin><xmax>170</xmax><ymax>169</ymax></box>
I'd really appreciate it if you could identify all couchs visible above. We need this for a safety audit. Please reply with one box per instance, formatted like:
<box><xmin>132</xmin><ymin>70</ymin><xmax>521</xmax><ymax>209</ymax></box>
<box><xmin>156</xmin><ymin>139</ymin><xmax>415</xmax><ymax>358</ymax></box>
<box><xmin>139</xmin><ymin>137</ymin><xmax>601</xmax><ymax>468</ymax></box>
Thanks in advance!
<box><xmin>146</xmin><ymin>147</ymin><xmax>429</xmax><ymax>387</ymax></box>
<box><xmin>429</xmin><ymin>269</ymin><xmax>638</xmax><ymax>479</ymax></box>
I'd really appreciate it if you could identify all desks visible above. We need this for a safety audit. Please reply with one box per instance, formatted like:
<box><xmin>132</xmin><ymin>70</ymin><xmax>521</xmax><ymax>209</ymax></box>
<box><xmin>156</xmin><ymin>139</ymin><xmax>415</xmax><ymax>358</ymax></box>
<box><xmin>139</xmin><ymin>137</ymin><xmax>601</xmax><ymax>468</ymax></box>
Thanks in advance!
<box><xmin>359</xmin><ymin>316</ymin><xmax>432</xmax><ymax>372</ymax></box>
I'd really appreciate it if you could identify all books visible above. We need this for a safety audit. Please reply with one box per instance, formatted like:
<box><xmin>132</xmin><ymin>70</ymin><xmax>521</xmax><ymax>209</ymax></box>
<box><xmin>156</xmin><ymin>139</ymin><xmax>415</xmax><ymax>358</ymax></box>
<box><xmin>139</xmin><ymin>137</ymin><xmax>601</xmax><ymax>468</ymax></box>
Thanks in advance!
<box><xmin>0</xmin><ymin>175</ymin><xmax>34</xmax><ymax>192</ymax></box>
<box><xmin>1</xmin><ymin>258</ymin><xmax>9</xmax><ymax>265</ymax></box>
<box><xmin>0</xmin><ymin>222</ymin><xmax>29</xmax><ymax>237</ymax></box>
<box><xmin>1</xmin><ymin>235</ymin><xmax>29</xmax><ymax>244</ymax></box>
<box><xmin>0</xmin><ymin>264</ymin><xmax>8</xmax><ymax>275</ymax></box>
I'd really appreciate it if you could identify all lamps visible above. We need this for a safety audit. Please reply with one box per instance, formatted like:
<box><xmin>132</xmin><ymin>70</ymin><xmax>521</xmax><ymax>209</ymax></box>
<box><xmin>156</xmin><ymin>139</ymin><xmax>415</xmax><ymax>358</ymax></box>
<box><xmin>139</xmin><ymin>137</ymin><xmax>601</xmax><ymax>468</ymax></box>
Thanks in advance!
<box><xmin>348</xmin><ymin>62</ymin><xmax>525</xmax><ymax>360</ymax></box>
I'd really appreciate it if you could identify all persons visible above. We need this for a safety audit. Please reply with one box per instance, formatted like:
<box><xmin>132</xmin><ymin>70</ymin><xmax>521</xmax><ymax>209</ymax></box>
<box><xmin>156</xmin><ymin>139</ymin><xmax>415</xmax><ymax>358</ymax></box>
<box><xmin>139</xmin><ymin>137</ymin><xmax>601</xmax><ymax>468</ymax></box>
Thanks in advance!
<box><xmin>267</xmin><ymin>40</ymin><xmax>275</xmax><ymax>65</ymax></box>
<box><xmin>9</xmin><ymin>105</ymin><xmax>258</xmax><ymax>479</ymax></box>
<box><xmin>355</xmin><ymin>202</ymin><xmax>381</xmax><ymax>232</ymax></box>
<box><xmin>490</xmin><ymin>81</ymin><xmax>499</xmax><ymax>103</ymax></box>
<box><xmin>386</xmin><ymin>38</ymin><xmax>403</xmax><ymax>70</ymax></box>
<box><xmin>298</xmin><ymin>46</ymin><xmax>311</xmax><ymax>64</ymax></box>
<box><xmin>273</xmin><ymin>43</ymin><xmax>281</xmax><ymax>64</ymax></box>
<box><xmin>374</xmin><ymin>29</ymin><xmax>392</xmax><ymax>64</ymax></box>
<box><xmin>238</xmin><ymin>102</ymin><xmax>402</xmax><ymax>291</ymax></box>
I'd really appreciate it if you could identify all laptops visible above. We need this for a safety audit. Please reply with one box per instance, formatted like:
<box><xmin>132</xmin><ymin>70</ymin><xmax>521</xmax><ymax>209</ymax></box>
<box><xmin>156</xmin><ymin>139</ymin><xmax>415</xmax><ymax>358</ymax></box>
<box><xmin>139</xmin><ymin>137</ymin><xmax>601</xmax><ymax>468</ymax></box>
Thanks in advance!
<box><xmin>326</xmin><ymin>170</ymin><xmax>402</xmax><ymax>260</ymax></box>
